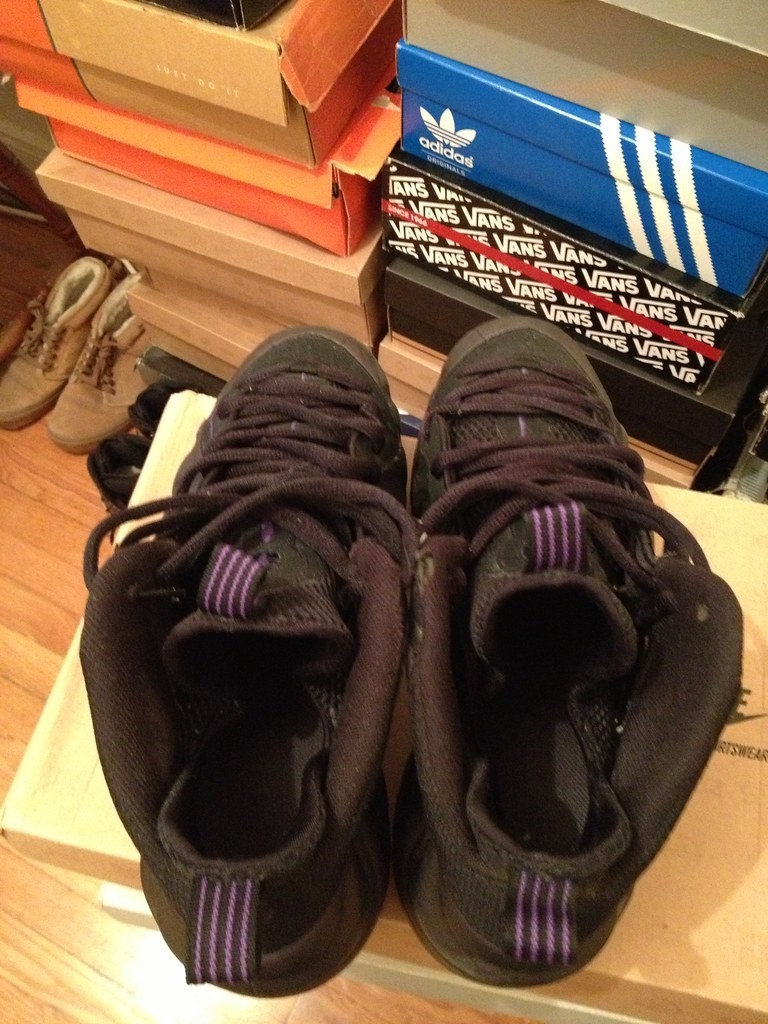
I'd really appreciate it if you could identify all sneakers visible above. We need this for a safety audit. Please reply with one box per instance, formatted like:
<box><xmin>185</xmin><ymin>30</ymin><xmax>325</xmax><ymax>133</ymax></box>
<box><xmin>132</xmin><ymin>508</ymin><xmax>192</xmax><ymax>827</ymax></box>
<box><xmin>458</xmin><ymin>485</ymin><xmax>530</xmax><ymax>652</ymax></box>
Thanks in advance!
<box><xmin>0</xmin><ymin>256</ymin><xmax>117</xmax><ymax>430</ymax></box>
<box><xmin>48</xmin><ymin>268</ymin><xmax>155</xmax><ymax>454</ymax></box>
<box><xmin>79</xmin><ymin>325</ymin><xmax>419</xmax><ymax>1000</ymax></box>
<box><xmin>405</xmin><ymin>316</ymin><xmax>744</xmax><ymax>986</ymax></box>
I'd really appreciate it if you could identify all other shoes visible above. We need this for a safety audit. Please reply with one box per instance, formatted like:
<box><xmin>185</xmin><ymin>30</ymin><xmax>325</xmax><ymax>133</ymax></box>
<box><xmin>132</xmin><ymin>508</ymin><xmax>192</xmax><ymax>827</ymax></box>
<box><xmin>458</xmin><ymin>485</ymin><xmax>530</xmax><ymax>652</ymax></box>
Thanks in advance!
<box><xmin>87</xmin><ymin>433</ymin><xmax>152</xmax><ymax>514</ymax></box>
<box><xmin>128</xmin><ymin>382</ymin><xmax>204</xmax><ymax>440</ymax></box>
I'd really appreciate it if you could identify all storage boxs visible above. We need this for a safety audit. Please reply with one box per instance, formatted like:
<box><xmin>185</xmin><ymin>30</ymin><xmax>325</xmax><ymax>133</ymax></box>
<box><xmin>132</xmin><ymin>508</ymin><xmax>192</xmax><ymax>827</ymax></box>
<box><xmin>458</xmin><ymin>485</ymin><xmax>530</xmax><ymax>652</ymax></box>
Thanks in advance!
<box><xmin>0</xmin><ymin>0</ymin><xmax>768</xmax><ymax>1024</ymax></box>
